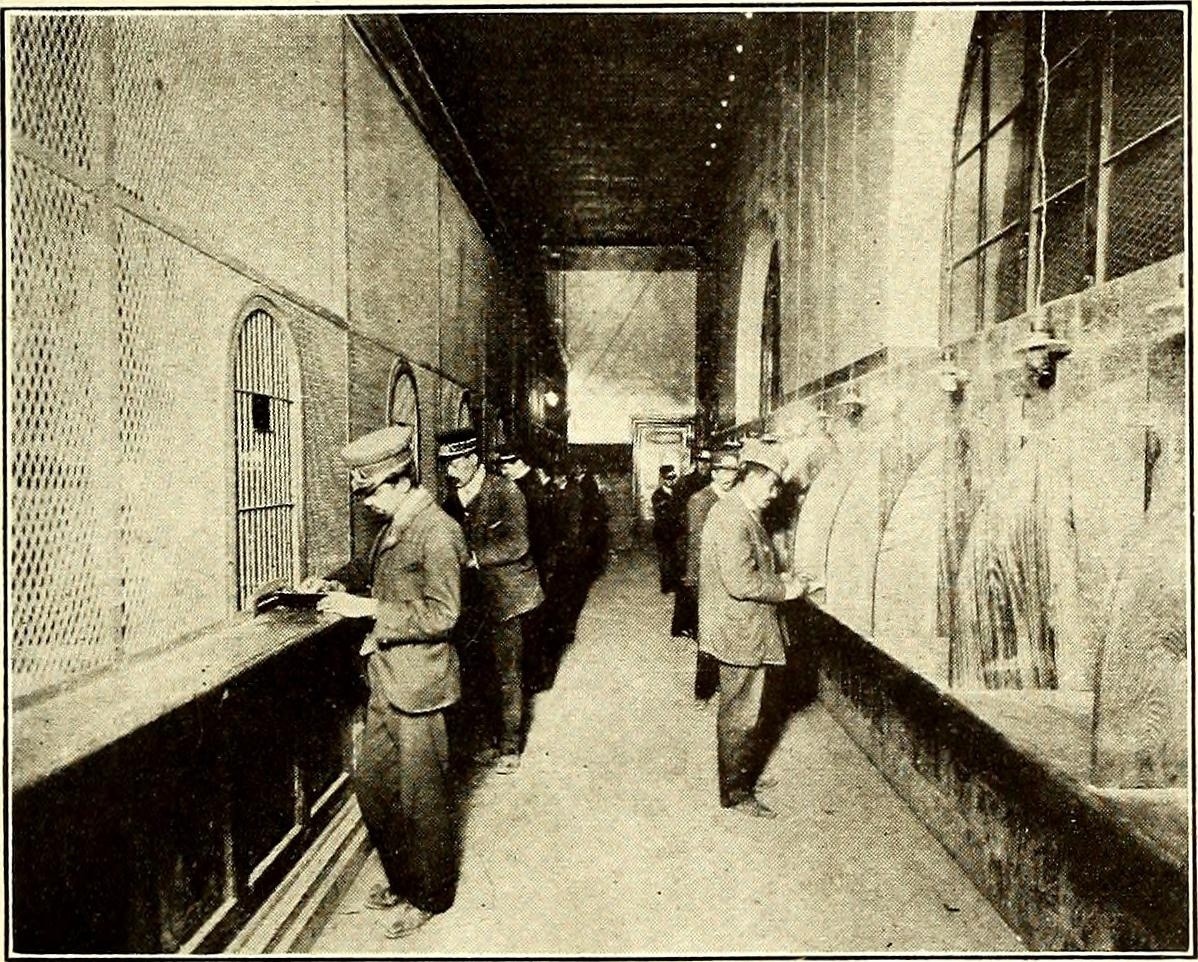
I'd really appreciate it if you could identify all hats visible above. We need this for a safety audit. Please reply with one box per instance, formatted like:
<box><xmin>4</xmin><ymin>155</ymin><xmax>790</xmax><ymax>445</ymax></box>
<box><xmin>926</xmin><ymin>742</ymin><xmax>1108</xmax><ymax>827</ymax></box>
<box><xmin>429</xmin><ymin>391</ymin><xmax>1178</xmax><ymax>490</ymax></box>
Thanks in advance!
<box><xmin>432</xmin><ymin>427</ymin><xmax>480</xmax><ymax>460</ymax></box>
<box><xmin>738</xmin><ymin>444</ymin><xmax>790</xmax><ymax>478</ymax></box>
<box><xmin>659</xmin><ymin>464</ymin><xmax>677</xmax><ymax>480</ymax></box>
<box><xmin>491</xmin><ymin>444</ymin><xmax>520</xmax><ymax>464</ymax></box>
<box><xmin>693</xmin><ymin>450</ymin><xmax>712</xmax><ymax>462</ymax></box>
<box><xmin>339</xmin><ymin>427</ymin><xmax>414</xmax><ymax>495</ymax></box>
<box><xmin>712</xmin><ymin>455</ymin><xmax>738</xmax><ymax>471</ymax></box>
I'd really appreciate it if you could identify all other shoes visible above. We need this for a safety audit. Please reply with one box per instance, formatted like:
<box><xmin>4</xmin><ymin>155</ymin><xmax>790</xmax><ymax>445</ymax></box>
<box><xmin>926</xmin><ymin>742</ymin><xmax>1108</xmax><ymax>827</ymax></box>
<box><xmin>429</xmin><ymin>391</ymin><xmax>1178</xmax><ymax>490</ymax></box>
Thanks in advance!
<box><xmin>756</xmin><ymin>775</ymin><xmax>779</xmax><ymax>793</ymax></box>
<box><xmin>474</xmin><ymin>740</ymin><xmax>496</xmax><ymax>763</ymax></box>
<box><xmin>695</xmin><ymin>697</ymin><xmax>707</xmax><ymax>712</ymax></box>
<box><xmin>682</xmin><ymin>628</ymin><xmax>698</xmax><ymax>638</ymax></box>
<box><xmin>364</xmin><ymin>886</ymin><xmax>406</xmax><ymax>910</ymax></box>
<box><xmin>734</xmin><ymin>797</ymin><xmax>778</xmax><ymax>819</ymax></box>
<box><xmin>387</xmin><ymin>907</ymin><xmax>433</xmax><ymax>939</ymax></box>
<box><xmin>496</xmin><ymin>754</ymin><xmax>520</xmax><ymax>776</ymax></box>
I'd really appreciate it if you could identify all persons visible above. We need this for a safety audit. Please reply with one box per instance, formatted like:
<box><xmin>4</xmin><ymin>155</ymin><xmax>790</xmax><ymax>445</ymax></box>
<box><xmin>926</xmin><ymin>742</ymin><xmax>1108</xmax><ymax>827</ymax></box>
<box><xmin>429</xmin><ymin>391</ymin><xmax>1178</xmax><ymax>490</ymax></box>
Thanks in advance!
<box><xmin>434</xmin><ymin>429</ymin><xmax>546</xmax><ymax>776</ymax></box>
<box><xmin>681</xmin><ymin>449</ymin><xmax>740</xmax><ymax>707</ymax></box>
<box><xmin>667</xmin><ymin>451</ymin><xmax>713</xmax><ymax>642</ymax></box>
<box><xmin>698</xmin><ymin>438</ymin><xmax>815</xmax><ymax>818</ymax></box>
<box><xmin>297</xmin><ymin>426</ymin><xmax>467</xmax><ymax>939</ymax></box>
<box><xmin>499</xmin><ymin>453</ymin><xmax>603</xmax><ymax>643</ymax></box>
<box><xmin>652</xmin><ymin>466</ymin><xmax>678</xmax><ymax>597</ymax></box>
